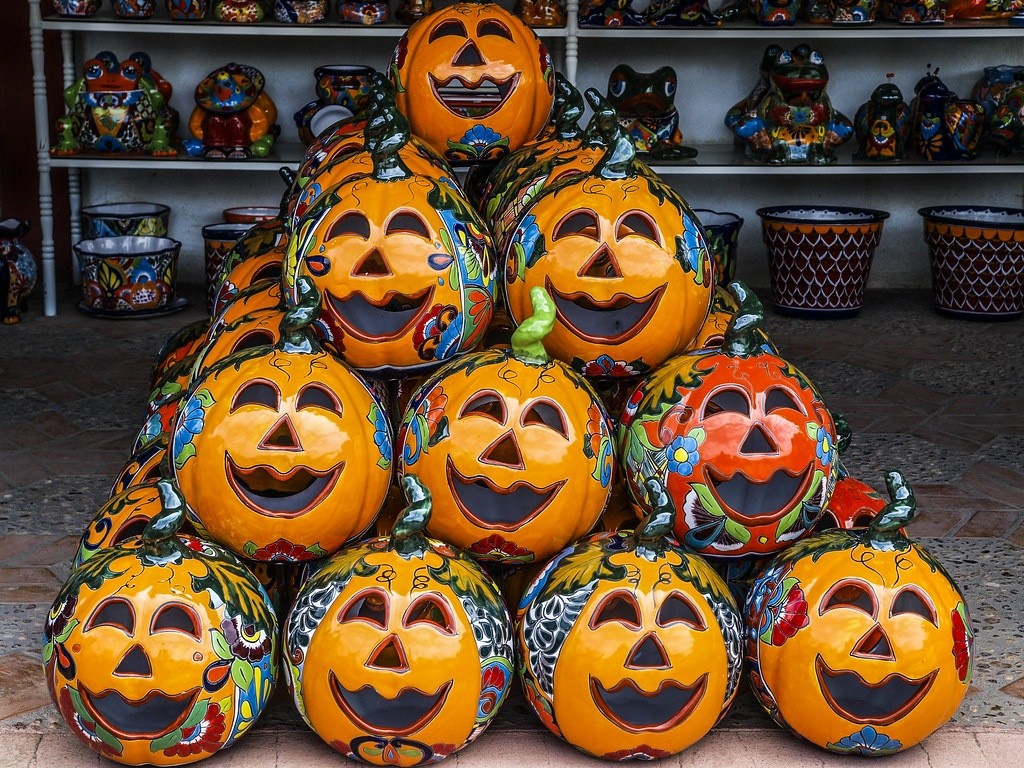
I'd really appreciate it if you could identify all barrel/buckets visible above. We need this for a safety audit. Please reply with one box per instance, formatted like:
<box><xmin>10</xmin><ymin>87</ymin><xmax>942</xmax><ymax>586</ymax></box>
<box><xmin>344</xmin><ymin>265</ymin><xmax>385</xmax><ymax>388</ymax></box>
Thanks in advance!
<box><xmin>689</xmin><ymin>207</ymin><xmax>744</xmax><ymax>295</ymax></box>
<box><xmin>756</xmin><ymin>205</ymin><xmax>892</xmax><ymax>316</ymax></box>
<box><xmin>71</xmin><ymin>200</ymin><xmax>182</xmax><ymax>313</ymax></box>
<box><xmin>202</xmin><ymin>205</ymin><xmax>283</xmax><ymax>317</ymax></box>
<box><xmin>917</xmin><ymin>204</ymin><xmax>1024</xmax><ymax>318</ymax></box>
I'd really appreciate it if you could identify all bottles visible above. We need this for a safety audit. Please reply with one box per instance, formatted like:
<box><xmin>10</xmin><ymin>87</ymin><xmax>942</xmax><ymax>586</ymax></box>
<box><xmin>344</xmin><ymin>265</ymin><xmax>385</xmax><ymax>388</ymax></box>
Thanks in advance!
<box><xmin>0</xmin><ymin>218</ymin><xmax>38</xmax><ymax>325</ymax></box>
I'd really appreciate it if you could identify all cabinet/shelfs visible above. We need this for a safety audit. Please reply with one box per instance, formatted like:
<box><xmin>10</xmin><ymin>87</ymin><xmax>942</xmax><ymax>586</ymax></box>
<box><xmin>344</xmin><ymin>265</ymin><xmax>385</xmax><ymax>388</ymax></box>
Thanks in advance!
<box><xmin>29</xmin><ymin>0</ymin><xmax>1024</xmax><ymax>317</ymax></box>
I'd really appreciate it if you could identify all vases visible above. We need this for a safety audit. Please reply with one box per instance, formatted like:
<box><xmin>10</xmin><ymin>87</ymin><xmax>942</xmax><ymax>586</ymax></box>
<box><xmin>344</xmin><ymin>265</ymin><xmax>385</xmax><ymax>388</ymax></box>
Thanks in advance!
<box><xmin>690</xmin><ymin>203</ymin><xmax>1024</xmax><ymax>318</ymax></box>
<box><xmin>0</xmin><ymin>218</ymin><xmax>38</xmax><ymax>325</ymax></box>
<box><xmin>73</xmin><ymin>198</ymin><xmax>280</xmax><ymax>317</ymax></box>
